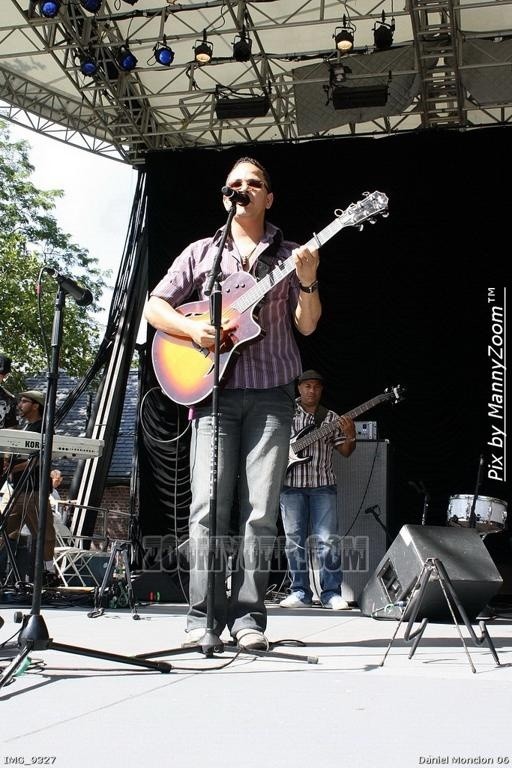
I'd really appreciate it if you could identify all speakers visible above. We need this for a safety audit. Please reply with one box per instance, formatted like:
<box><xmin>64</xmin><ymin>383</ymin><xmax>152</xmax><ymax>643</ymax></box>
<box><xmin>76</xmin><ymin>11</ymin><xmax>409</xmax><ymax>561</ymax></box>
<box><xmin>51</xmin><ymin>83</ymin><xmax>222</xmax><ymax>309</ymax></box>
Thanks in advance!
<box><xmin>309</xmin><ymin>440</ymin><xmax>387</xmax><ymax>605</ymax></box>
<box><xmin>332</xmin><ymin>83</ymin><xmax>389</xmax><ymax>111</ymax></box>
<box><xmin>358</xmin><ymin>526</ymin><xmax>503</xmax><ymax>619</ymax></box>
<box><xmin>216</xmin><ymin>96</ymin><xmax>271</xmax><ymax>120</ymax></box>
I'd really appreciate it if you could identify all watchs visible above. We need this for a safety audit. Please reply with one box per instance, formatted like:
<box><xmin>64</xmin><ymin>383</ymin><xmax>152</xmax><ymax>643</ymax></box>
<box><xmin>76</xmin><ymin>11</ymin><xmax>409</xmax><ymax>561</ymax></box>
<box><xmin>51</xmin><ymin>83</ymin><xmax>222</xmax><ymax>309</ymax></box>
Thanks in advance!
<box><xmin>299</xmin><ymin>279</ymin><xmax>319</xmax><ymax>294</ymax></box>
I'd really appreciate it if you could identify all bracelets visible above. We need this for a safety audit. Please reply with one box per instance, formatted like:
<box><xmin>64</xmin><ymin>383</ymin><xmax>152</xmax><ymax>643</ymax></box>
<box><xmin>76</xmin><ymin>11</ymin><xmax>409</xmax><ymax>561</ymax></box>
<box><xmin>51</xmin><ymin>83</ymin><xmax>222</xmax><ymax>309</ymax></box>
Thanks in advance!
<box><xmin>345</xmin><ymin>433</ymin><xmax>357</xmax><ymax>443</ymax></box>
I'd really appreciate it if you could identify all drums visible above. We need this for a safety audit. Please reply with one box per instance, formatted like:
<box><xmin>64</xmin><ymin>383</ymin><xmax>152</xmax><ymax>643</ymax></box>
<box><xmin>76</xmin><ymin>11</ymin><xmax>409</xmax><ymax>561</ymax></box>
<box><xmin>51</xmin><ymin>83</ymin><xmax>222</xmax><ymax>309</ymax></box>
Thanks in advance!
<box><xmin>447</xmin><ymin>495</ymin><xmax>507</xmax><ymax>533</ymax></box>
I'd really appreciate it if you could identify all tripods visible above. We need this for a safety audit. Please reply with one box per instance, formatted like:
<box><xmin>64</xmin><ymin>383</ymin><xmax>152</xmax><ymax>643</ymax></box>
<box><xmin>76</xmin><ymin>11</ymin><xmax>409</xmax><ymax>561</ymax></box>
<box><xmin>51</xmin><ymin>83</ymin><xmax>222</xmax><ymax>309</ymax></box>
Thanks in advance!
<box><xmin>129</xmin><ymin>208</ymin><xmax>318</xmax><ymax>664</ymax></box>
<box><xmin>0</xmin><ymin>307</ymin><xmax>171</xmax><ymax>690</ymax></box>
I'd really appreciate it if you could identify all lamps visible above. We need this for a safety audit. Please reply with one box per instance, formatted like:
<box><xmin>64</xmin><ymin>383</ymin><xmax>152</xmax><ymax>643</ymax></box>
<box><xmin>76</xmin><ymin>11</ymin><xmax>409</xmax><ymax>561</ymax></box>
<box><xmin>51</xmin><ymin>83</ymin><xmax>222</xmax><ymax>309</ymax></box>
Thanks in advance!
<box><xmin>114</xmin><ymin>38</ymin><xmax>138</xmax><ymax>71</ymax></box>
<box><xmin>80</xmin><ymin>0</ymin><xmax>104</xmax><ymax>13</ymax></box>
<box><xmin>192</xmin><ymin>28</ymin><xmax>213</xmax><ymax>63</ymax></box>
<box><xmin>231</xmin><ymin>24</ymin><xmax>252</xmax><ymax>62</ymax></box>
<box><xmin>146</xmin><ymin>35</ymin><xmax>175</xmax><ymax>67</ymax></box>
<box><xmin>41</xmin><ymin>1</ymin><xmax>60</xmax><ymax>18</ymax></box>
<box><xmin>373</xmin><ymin>10</ymin><xmax>396</xmax><ymax>50</ymax></box>
<box><xmin>81</xmin><ymin>43</ymin><xmax>100</xmax><ymax>77</ymax></box>
<box><xmin>332</xmin><ymin>14</ymin><xmax>356</xmax><ymax>53</ymax></box>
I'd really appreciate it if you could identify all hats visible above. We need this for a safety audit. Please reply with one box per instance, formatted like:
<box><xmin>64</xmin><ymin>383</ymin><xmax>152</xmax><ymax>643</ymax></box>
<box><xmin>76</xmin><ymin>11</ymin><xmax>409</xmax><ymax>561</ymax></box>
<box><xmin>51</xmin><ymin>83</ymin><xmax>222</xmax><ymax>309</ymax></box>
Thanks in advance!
<box><xmin>299</xmin><ymin>370</ymin><xmax>322</xmax><ymax>382</ymax></box>
<box><xmin>18</xmin><ymin>390</ymin><xmax>45</xmax><ymax>405</ymax></box>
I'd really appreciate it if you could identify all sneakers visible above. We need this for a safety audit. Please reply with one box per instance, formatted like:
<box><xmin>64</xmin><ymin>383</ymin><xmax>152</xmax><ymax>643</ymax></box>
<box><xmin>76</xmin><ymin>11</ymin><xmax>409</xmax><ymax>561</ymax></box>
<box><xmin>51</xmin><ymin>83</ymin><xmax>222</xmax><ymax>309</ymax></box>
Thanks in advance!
<box><xmin>236</xmin><ymin>627</ymin><xmax>267</xmax><ymax>649</ymax></box>
<box><xmin>280</xmin><ymin>591</ymin><xmax>312</xmax><ymax>607</ymax></box>
<box><xmin>324</xmin><ymin>595</ymin><xmax>348</xmax><ymax>609</ymax></box>
<box><xmin>181</xmin><ymin>627</ymin><xmax>206</xmax><ymax>647</ymax></box>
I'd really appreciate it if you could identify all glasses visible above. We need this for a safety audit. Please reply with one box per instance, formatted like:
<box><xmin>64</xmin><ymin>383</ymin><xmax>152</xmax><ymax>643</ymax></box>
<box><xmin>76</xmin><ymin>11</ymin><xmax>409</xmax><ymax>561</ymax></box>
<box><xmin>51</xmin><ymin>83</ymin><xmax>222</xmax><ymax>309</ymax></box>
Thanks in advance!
<box><xmin>228</xmin><ymin>180</ymin><xmax>269</xmax><ymax>194</ymax></box>
<box><xmin>19</xmin><ymin>399</ymin><xmax>36</xmax><ymax>403</ymax></box>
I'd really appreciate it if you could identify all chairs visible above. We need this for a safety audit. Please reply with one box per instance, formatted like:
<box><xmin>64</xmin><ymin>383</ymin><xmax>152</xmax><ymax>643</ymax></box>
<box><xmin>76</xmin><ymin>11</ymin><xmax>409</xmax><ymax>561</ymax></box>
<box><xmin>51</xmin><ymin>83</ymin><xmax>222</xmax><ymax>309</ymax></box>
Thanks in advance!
<box><xmin>52</xmin><ymin>525</ymin><xmax>99</xmax><ymax>586</ymax></box>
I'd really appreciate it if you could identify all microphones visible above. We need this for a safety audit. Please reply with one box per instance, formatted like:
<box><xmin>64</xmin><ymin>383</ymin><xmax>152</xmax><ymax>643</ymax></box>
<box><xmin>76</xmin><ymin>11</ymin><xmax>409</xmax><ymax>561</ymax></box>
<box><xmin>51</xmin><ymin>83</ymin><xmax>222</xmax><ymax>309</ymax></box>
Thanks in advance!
<box><xmin>222</xmin><ymin>186</ymin><xmax>251</xmax><ymax>207</ymax></box>
<box><xmin>365</xmin><ymin>505</ymin><xmax>378</xmax><ymax>514</ymax></box>
<box><xmin>46</xmin><ymin>268</ymin><xmax>93</xmax><ymax>306</ymax></box>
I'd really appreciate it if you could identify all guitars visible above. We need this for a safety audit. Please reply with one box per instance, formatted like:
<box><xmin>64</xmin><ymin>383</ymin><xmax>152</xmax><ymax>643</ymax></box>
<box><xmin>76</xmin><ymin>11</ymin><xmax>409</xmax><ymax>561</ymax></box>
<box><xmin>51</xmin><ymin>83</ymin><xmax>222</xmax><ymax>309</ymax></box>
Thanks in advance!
<box><xmin>286</xmin><ymin>386</ymin><xmax>404</xmax><ymax>474</ymax></box>
<box><xmin>152</xmin><ymin>187</ymin><xmax>394</xmax><ymax>406</ymax></box>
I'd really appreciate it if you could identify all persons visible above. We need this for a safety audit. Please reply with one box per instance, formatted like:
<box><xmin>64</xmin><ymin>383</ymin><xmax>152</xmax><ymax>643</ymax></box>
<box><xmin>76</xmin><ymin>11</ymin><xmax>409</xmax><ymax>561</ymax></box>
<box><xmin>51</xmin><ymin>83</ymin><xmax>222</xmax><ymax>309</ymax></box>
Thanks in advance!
<box><xmin>3</xmin><ymin>390</ymin><xmax>61</xmax><ymax>587</ymax></box>
<box><xmin>0</xmin><ymin>351</ymin><xmax>17</xmax><ymax>490</ymax></box>
<box><xmin>49</xmin><ymin>468</ymin><xmax>64</xmax><ymax>500</ymax></box>
<box><xmin>145</xmin><ymin>156</ymin><xmax>322</xmax><ymax>650</ymax></box>
<box><xmin>278</xmin><ymin>370</ymin><xmax>357</xmax><ymax>610</ymax></box>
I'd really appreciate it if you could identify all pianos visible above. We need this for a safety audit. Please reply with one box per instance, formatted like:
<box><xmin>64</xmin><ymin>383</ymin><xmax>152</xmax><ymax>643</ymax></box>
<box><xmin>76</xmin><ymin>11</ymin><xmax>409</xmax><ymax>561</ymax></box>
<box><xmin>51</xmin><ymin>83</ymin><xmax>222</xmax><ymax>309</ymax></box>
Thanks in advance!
<box><xmin>0</xmin><ymin>426</ymin><xmax>101</xmax><ymax>460</ymax></box>
